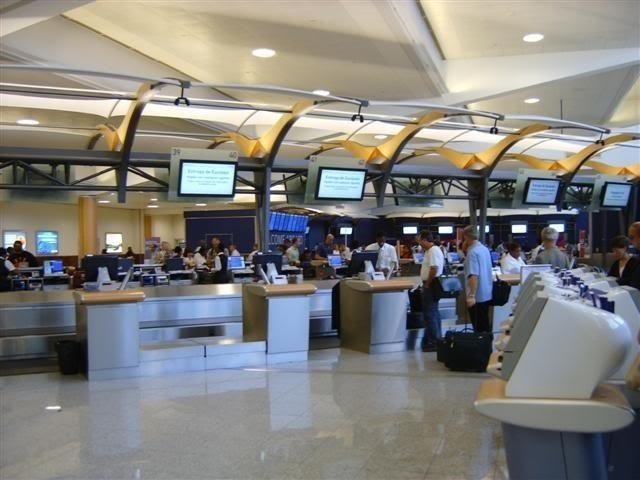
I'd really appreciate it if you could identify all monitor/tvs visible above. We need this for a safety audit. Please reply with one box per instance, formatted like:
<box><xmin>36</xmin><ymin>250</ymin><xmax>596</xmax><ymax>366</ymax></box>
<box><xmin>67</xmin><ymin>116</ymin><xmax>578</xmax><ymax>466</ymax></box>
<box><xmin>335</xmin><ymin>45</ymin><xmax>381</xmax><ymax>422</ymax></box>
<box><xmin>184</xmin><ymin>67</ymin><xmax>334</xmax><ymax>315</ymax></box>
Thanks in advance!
<box><xmin>253</xmin><ymin>254</ymin><xmax>283</xmax><ymax>283</ymax></box>
<box><xmin>316</xmin><ymin>167</ymin><xmax>368</xmax><ymax>202</ymax></box>
<box><xmin>349</xmin><ymin>251</ymin><xmax>379</xmax><ymax>280</ymax></box>
<box><xmin>599</xmin><ymin>181</ymin><xmax>634</xmax><ymax>209</ymax></box>
<box><xmin>522</xmin><ymin>176</ymin><xmax>562</xmax><ymax>206</ymax></box>
<box><xmin>227</xmin><ymin>256</ymin><xmax>246</xmax><ymax>271</ymax></box>
<box><xmin>43</xmin><ymin>258</ymin><xmax>64</xmax><ymax>277</ymax></box>
<box><xmin>118</xmin><ymin>259</ymin><xmax>133</xmax><ymax>273</ymax></box>
<box><xmin>85</xmin><ymin>255</ymin><xmax>118</xmax><ymax>282</ymax></box>
<box><xmin>176</xmin><ymin>158</ymin><xmax>239</xmax><ymax>198</ymax></box>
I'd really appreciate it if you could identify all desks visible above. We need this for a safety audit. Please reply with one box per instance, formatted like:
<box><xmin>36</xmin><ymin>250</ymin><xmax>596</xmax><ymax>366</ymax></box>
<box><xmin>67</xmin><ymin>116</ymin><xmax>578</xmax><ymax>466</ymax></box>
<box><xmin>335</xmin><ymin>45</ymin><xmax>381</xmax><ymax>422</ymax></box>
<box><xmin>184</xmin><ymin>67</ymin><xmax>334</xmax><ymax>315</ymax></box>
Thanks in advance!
<box><xmin>242</xmin><ymin>283</ymin><xmax>316</xmax><ymax>365</ymax></box>
<box><xmin>73</xmin><ymin>290</ymin><xmax>146</xmax><ymax>381</ymax></box>
<box><xmin>340</xmin><ymin>279</ymin><xmax>417</xmax><ymax>356</ymax></box>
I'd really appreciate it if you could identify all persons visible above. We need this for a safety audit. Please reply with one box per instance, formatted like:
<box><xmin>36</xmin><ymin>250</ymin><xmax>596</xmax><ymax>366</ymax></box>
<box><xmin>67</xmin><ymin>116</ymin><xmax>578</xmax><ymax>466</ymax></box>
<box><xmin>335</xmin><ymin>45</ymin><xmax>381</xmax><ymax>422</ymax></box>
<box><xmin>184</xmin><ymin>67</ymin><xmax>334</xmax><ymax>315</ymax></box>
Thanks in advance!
<box><xmin>366</xmin><ymin>232</ymin><xmax>399</xmax><ymax>276</ymax></box>
<box><xmin>0</xmin><ymin>240</ymin><xmax>19</xmax><ymax>293</ymax></box>
<box><xmin>418</xmin><ymin>230</ymin><xmax>445</xmax><ymax>350</ymax></box>
<box><xmin>123</xmin><ymin>246</ymin><xmax>136</xmax><ymax>264</ymax></box>
<box><xmin>494</xmin><ymin>234</ymin><xmax>511</xmax><ymax>256</ymax></box>
<box><xmin>150</xmin><ymin>244</ymin><xmax>158</xmax><ymax>260</ymax></box>
<box><xmin>248</xmin><ymin>243</ymin><xmax>259</xmax><ymax>261</ymax></box>
<box><xmin>435</xmin><ymin>240</ymin><xmax>449</xmax><ymax>275</ymax></box>
<box><xmin>463</xmin><ymin>226</ymin><xmax>494</xmax><ymax>331</ymax></box>
<box><xmin>536</xmin><ymin>227</ymin><xmax>570</xmax><ymax>268</ymax></box>
<box><xmin>607</xmin><ymin>236</ymin><xmax>637</xmax><ymax>287</ymax></box>
<box><xmin>501</xmin><ymin>243</ymin><xmax>527</xmax><ymax>317</ymax></box>
<box><xmin>100</xmin><ymin>249</ymin><xmax>109</xmax><ymax>255</ymax></box>
<box><xmin>161</xmin><ymin>236</ymin><xmax>242</xmax><ymax>284</ymax></box>
<box><xmin>627</xmin><ymin>222</ymin><xmax>640</xmax><ymax>272</ymax></box>
<box><xmin>530</xmin><ymin>237</ymin><xmax>544</xmax><ymax>264</ymax></box>
<box><xmin>275</xmin><ymin>234</ymin><xmax>364</xmax><ymax>278</ymax></box>
<box><xmin>9</xmin><ymin>241</ymin><xmax>38</xmax><ymax>291</ymax></box>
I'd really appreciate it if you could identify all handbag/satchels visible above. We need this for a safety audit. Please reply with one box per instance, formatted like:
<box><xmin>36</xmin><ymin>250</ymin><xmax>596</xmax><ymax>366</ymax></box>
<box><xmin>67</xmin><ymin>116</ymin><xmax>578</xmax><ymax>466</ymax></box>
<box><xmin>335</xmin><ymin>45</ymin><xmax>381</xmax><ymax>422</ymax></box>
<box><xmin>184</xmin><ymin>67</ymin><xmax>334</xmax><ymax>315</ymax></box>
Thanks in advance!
<box><xmin>489</xmin><ymin>250</ymin><xmax>511</xmax><ymax>306</ymax></box>
<box><xmin>434</xmin><ymin>272</ymin><xmax>463</xmax><ymax>298</ymax></box>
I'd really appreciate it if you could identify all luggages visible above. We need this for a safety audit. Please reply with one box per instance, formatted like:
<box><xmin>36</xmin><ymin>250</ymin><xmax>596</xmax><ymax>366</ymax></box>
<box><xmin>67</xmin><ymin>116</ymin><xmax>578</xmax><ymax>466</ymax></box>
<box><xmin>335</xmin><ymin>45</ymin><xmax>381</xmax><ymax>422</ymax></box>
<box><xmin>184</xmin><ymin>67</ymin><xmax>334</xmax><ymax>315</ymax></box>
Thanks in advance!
<box><xmin>443</xmin><ymin>327</ymin><xmax>493</xmax><ymax>371</ymax></box>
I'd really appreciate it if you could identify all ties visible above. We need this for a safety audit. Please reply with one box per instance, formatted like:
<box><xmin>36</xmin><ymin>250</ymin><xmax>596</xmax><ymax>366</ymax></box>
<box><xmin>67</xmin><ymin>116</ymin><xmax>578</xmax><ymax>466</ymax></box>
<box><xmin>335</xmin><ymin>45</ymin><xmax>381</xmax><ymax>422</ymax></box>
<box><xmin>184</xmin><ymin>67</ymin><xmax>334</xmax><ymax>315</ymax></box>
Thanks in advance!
<box><xmin>377</xmin><ymin>248</ymin><xmax>381</xmax><ymax>270</ymax></box>
